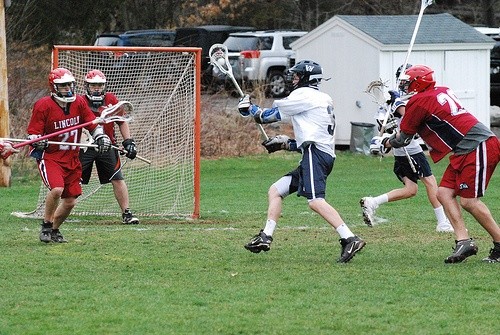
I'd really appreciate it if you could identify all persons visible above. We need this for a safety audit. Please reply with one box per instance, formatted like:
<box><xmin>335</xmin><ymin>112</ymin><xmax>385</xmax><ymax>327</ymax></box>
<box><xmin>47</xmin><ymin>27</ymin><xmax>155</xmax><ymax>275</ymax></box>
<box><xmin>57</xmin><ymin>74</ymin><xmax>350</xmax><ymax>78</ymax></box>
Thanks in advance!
<box><xmin>236</xmin><ymin>60</ymin><xmax>368</xmax><ymax>263</ymax></box>
<box><xmin>0</xmin><ymin>140</ymin><xmax>20</xmax><ymax>160</ymax></box>
<box><xmin>77</xmin><ymin>69</ymin><xmax>140</xmax><ymax>224</ymax></box>
<box><xmin>370</xmin><ymin>65</ymin><xmax>500</xmax><ymax>264</ymax></box>
<box><xmin>358</xmin><ymin>62</ymin><xmax>469</xmax><ymax>233</ymax></box>
<box><xmin>0</xmin><ymin>67</ymin><xmax>112</xmax><ymax>243</ymax></box>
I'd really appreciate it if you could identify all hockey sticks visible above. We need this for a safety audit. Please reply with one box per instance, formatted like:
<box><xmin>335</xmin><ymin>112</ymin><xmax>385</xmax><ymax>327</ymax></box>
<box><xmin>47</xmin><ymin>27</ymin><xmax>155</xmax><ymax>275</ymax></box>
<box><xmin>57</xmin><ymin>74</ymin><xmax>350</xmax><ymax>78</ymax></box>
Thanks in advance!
<box><xmin>0</xmin><ymin>138</ymin><xmax>98</xmax><ymax>148</ymax></box>
<box><xmin>81</xmin><ymin>127</ymin><xmax>151</xmax><ymax>165</ymax></box>
<box><xmin>13</xmin><ymin>101</ymin><xmax>135</xmax><ymax>148</ymax></box>
<box><xmin>365</xmin><ymin>79</ymin><xmax>424</xmax><ymax>180</ymax></box>
<box><xmin>208</xmin><ymin>44</ymin><xmax>269</xmax><ymax>141</ymax></box>
<box><xmin>379</xmin><ymin>0</ymin><xmax>433</xmax><ymax>137</ymax></box>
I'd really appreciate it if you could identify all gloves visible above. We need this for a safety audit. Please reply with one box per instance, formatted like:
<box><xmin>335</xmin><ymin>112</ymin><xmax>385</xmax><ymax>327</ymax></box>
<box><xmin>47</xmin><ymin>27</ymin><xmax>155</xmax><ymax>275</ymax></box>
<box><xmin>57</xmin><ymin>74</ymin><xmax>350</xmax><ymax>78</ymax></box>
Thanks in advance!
<box><xmin>119</xmin><ymin>139</ymin><xmax>137</xmax><ymax>159</ymax></box>
<box><xmin>237</xmin><ymin>94</ymin><xmax>251</xmax><ymax>117</ymax></box>
<box><xmin>262</xmin><ymin>134</ymin><xmax>290</xmax><ymax>153</ymax></box>
<box><xmin>386</xmin><ymin>90</ymin><xmax>406</xmax><ymax>110</ymax></box>
<box><xmin>370</xmin><ymin>136</ymin><xmax>392</xmax><ymax>154</ymax></box>
<box><xmin>0</xmin><ymin>141</ymin><xmax>19</xmax><ymax>159</ymax></box>
<box><xmin>28</xmin><ymin>134</ymin><xmax>48</xmax><ymax>149</ymax></box>
<box><xmin>94</xmin><ymin>134</ymin><xmax>111</xmax><ymax>155</ymax></box>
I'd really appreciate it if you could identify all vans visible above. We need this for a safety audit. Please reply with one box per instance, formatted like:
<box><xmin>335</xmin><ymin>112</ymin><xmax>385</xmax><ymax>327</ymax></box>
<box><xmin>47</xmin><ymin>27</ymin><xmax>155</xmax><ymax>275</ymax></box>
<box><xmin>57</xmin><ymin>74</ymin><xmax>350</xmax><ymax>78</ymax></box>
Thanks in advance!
<box><xmin>80</xmin><ymin>28</ymin><xmax>176</xmax><ymax>94</ymax></box>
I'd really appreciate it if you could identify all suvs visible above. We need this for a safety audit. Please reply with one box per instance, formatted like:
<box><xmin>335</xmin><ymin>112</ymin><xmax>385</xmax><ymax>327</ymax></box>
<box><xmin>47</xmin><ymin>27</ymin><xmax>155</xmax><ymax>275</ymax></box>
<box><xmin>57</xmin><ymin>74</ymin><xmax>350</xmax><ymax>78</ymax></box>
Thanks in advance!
<box><xmin>212</xmin><ymin>30</ymin><xmax>312</xmax><ymax>98</ymax></box>
<box><xmin>170</xmin><ymin>25</ymin><xmax>262</xmax><ymax>95</ymax></box>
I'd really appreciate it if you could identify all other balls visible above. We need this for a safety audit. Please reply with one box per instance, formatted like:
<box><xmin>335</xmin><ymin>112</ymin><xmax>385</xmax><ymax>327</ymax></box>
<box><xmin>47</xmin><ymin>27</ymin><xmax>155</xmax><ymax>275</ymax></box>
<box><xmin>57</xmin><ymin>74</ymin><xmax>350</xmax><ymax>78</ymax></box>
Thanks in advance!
<box><xmin>218</xmin><ymin>57</ymin><xmax>225</xmax><ymax>66</ymax></box>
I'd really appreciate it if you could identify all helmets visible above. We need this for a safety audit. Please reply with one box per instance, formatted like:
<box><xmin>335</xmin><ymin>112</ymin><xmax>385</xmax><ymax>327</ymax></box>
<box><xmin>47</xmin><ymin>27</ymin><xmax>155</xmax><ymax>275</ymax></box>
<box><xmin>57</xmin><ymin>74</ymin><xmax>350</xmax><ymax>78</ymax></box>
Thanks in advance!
<box><xmin>84</xmin><ymin>69</ymin><xmax>106</xmax><ymax>102</ymax></box>
<box><xmin>399</xmin><ymin>65</ymin><xmax>434</xmax><ymax>96</ymax></box>
<box><xmin>396</xmin><ymin>64</ymin><xmax>411</xmax><ymax>84</ymax></box>
<box><xmin>287</xmin><ymin>60</ymin><xmax>321</xmax><ymax>88</ymax></box>
<box><xmin>48</xmin><ymin>68</ymin><xmax>76</xmax><ymax>103</ymax></box>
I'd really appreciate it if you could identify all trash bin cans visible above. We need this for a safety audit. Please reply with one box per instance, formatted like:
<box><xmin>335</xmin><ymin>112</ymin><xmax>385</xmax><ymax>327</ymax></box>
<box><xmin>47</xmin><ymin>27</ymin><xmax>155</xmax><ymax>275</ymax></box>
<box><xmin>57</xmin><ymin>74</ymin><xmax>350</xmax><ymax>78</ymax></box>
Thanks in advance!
<box><xmin>350</xmin><ymin>121</ymin><xmax>376</xmax><ymax>154</ymax></box>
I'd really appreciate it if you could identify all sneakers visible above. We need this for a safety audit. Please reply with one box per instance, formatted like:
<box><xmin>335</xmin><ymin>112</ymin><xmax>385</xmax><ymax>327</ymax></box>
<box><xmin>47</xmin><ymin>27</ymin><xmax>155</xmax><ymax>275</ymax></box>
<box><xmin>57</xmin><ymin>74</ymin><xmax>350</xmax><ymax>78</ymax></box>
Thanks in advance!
<box><xmin>243</xmin><ymin>229</ymin><xmax>272</xmax><ymax>253</ymax></box>
<box><xmin>360</xmin><ymin>196</ymin><xmax>376</xmax><ymax>226</ymax></box>
<box><xmin>39</xmin><ymin>219</ymin><xmax>52</xmax><ymax>242</ymax></box>
<box><xmin>436</xmin><ymin>222</ymin><xmax>455</xmax><ymax>233</ymax></box>
<box><xmin>336</xmin><ymin>235</ymin><xmax>366</xmax><ymax>263</ymax></box>
<box><xmin>51</xmin><ymin>229</ymin><xmax>67</xmax><ymax>243</ymax></box>
<box><xmin>483</xmin><ymin>242</ymin><xmax>500</xmax><ymax>262</ymax></box>
<box><xmin>122</xmin><ymin>209</ymin><xmax>139</xmax><ymax>224</ymax></box>
<box><xmin>445</xmin><ymin>238</ymin><xmax>478</xmax><ymax>263</ymax></box>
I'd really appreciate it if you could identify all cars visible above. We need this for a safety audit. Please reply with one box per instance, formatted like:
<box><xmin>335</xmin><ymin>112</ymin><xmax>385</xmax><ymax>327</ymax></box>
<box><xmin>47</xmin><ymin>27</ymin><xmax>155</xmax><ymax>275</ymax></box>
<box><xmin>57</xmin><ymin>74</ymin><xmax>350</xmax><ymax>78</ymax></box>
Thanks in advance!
<box><xmin>473</xmin><ymin>26</ymin><xmax>499</xmax><ymax>106</ymax></box>
